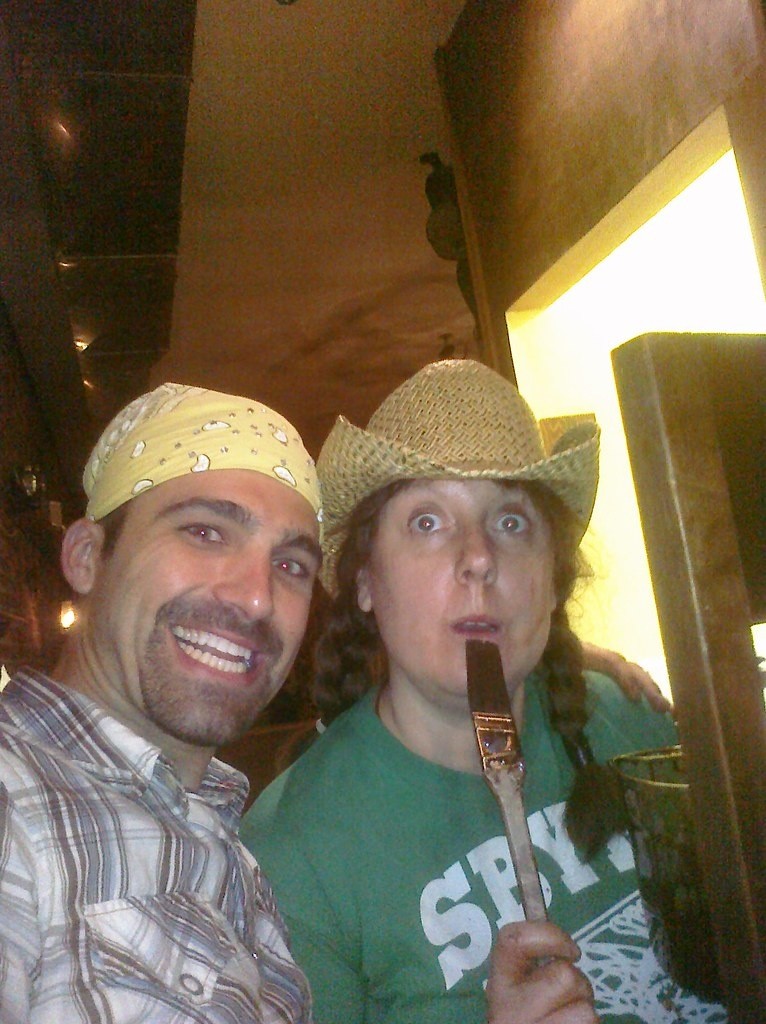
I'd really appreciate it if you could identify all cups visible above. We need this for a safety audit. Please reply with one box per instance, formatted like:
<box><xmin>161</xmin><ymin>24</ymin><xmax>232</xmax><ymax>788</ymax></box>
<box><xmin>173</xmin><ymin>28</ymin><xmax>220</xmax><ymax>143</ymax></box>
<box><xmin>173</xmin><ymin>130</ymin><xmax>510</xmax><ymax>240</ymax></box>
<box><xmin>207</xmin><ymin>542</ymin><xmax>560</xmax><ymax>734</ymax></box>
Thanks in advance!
<box><xmin>611</xmin><ymin>742</ymin><xmax>717</xmax><ymax>1001</ymax></box>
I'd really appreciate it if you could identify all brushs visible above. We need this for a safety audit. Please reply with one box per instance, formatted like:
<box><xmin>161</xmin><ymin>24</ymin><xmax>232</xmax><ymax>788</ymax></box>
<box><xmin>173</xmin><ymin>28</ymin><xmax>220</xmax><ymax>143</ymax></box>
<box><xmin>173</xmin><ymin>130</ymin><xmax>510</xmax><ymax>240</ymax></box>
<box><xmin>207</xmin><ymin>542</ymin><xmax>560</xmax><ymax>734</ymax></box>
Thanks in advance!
<box><xmin>465</xmin><ymin>638</ymin><xmax>547</xmax><ymax>921</ymax></box>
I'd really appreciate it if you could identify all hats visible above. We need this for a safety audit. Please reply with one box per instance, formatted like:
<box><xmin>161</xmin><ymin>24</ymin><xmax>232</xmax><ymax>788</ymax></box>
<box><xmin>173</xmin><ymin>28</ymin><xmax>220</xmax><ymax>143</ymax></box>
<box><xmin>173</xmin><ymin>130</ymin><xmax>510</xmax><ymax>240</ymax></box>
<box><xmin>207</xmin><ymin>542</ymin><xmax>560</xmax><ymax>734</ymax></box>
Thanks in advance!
<box><xmin>317</xmin><ymin>359</ymin><xmax>603</xmax><ymax>600</ymax></box>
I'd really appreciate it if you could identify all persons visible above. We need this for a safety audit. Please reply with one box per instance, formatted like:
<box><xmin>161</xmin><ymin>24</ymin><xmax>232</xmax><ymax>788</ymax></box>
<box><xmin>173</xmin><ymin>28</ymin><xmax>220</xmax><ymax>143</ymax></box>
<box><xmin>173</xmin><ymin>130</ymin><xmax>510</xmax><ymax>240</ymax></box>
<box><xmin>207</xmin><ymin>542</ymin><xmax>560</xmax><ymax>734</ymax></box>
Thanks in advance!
<box><xmin>239</xmin><ymin>361</ymin><xmax>723</xmax><ymax>1024</ymax></box>
<box><xmin>0</xmin><ymin>380</ymin><xmax>672</xmax><ymax>1024</ymax></box>
